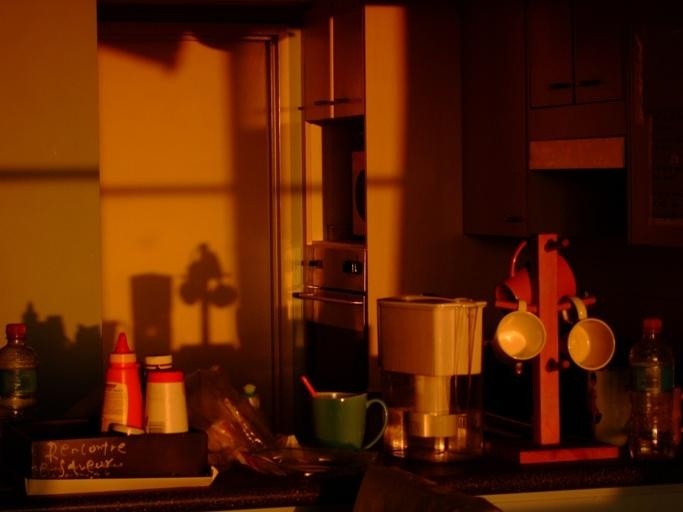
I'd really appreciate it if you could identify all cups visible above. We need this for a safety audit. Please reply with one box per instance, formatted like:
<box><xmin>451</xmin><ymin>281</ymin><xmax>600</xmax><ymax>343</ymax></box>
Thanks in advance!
<box><xmin>311</xmin><ymin>392</ymin><xmax>389</xmax><ymax>456</ymax></box>
<box><xmin>562</xmin><ymin>296</ymin><xmax>616</xmax><ymax>373</ymax></box>
<box><xmin>490</xmin><ymin>298</ymin><xmax>550</xmax><ymax>375</ymax></box>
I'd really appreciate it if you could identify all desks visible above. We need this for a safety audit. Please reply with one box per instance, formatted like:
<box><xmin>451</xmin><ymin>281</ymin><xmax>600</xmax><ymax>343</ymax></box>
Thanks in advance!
<box><xmin>0</xmin><ymin>428</ymin><xmax>683</xmax><ymax>512</ymax></box>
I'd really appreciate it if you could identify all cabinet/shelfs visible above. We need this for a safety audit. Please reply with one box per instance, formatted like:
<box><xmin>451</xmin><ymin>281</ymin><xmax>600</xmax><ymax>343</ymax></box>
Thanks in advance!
<box><xmin>295</xmin><ymin>2</ymin><xmax>459</xmax><ymax>120</ymax></box>
<box><xmin>524</xmin><ymin>0</ymin><xmax>626</xmax><ymax>137</ymax></box>
<box><xmin>454</xmin><ymin>1</ymin><xmax>580</xmax><ymax>240</ymax></box>
<box><xmin>625</xmin><ymin>0</ymin><xmax>683</xmax><ymax>244</ymax></box>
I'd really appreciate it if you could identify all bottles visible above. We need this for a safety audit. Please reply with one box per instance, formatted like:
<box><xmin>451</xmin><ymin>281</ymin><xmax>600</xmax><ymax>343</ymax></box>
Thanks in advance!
<box><xmin>142</xmin><ymin>353</ymin><xmax>191</xmax><ymax>436</ymax></box>
<box><xmin>630</xmin><ymin>318</ymin><xmax>674</xmax><ymax>462</ymax></box>
<box><xmin>97</xmin><ymin>330</ymin><xmax>146</xmax><ymax>441</ymax></box>
<box><xmin>1</xmin><ymin>320</ymin><xmax>47</xmax><ymax>448</ymax></box>
